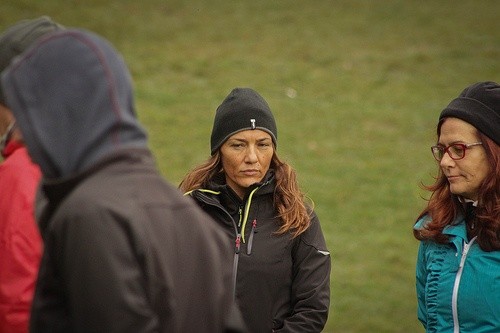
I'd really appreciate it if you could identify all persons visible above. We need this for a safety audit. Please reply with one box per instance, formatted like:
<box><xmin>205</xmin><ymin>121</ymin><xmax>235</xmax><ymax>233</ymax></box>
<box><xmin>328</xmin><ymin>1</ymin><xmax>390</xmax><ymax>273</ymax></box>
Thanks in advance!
<box><xmin>412</xmin><ymin>82</ymin><xmax>500</xmax><ymax>333</ymax></box>
<box><xmin>178</xmin><ymin>88</ymin><xmax>331</xmax><ymax>333</ymax></box>
<box><xmin>0</xmin><ymin>17</ymin><xmax>248</xmax><ymax>333</ymax></box>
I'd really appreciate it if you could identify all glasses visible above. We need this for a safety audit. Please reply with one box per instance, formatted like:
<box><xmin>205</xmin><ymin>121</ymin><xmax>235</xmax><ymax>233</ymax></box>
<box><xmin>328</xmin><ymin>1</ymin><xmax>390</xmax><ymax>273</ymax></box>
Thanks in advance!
<box><xmin>431</xmin><ymin>142</ymin><xmax>483</xmax><ymax>162</ymax></box>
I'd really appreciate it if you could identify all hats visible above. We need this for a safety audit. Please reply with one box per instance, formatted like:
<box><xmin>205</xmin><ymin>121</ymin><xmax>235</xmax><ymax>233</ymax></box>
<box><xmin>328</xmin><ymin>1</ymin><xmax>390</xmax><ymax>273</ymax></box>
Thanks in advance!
<box><xmin>437</xmin><ymin>81</ymin><xmax>500</xmax><ymax>146</ymax></box>
<box><xmin>0</xmin><ymin>15</ymin><xmax>68</xmax><ymax>108</ymax></box>
<box><xmin>210</xmin><ymin>88</ymin><xmax>277</xmax><ymax>156</ymax></box>
